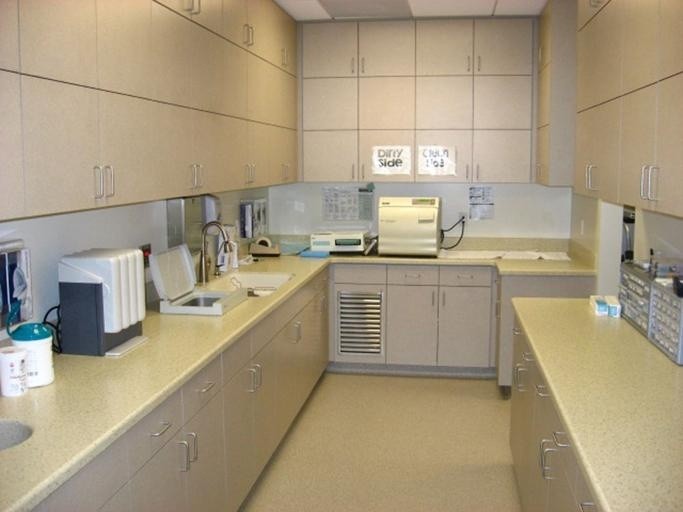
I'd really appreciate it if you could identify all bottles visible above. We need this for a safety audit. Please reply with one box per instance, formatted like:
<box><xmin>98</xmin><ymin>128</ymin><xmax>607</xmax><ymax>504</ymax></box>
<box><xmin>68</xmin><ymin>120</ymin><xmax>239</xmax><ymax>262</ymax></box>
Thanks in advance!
<box><xmin>10</xmin><ymin>323</ymin><xmax>54</xmax><ymax>389</ymax></box>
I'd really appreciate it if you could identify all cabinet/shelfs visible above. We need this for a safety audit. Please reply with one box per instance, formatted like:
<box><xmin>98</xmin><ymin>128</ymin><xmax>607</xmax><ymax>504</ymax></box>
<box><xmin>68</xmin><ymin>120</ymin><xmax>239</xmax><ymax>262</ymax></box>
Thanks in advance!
<box><xmin>507</xmin><ymin>299</ymin><xmax>604</xmax><ymax>512</ymax></box>
<box><xmin>329</xmin><ymin>264</ymin><xmax>494</xmax><ymax>383</ymax></box>
<box><xmin>2</xmin><ymin>2</ymin><xmax>300</xmax><ymax>224</ymax></box>
<box><xmin>573</xmin><ymin>1</ymin><xmax>682</xmax><ymax>220</ymax></box>
<box><xmin>28</xmin><ymin>264</ymin><xmax>329</xmax><ymax>512</ymax></box>
<box><xmin>297</xmin><ymin>0</ymin><xmax>572</xmax><ymax>185</ymax></box>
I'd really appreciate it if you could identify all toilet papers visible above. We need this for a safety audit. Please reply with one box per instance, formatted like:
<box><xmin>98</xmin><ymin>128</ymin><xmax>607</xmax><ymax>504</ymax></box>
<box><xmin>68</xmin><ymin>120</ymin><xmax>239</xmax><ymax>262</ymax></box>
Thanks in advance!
<box><xmin>604</xmin><ymin>294</ymin><xmax>621</xmax><ymax>318</ymax></box>
<box><xmin>589</xmin><ymin>294</ymin><xmax>609</xmax><ymax>316</ymax></box>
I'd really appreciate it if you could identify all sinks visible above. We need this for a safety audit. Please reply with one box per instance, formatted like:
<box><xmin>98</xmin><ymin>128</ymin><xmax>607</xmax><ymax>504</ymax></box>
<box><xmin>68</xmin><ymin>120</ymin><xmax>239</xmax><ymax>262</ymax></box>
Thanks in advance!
<box><xmin>209</xmin><ymin>271</ymin><xmax>294</xmax><ymax>298</ymax></box>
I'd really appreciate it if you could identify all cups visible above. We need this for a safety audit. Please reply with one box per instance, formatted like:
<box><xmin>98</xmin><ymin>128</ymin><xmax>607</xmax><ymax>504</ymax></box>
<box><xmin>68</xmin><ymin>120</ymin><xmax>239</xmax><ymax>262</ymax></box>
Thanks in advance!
<box><xmin>0</xmin><ymin>346</ymin><xmax>28</xmax><ymax>397</ymax></box>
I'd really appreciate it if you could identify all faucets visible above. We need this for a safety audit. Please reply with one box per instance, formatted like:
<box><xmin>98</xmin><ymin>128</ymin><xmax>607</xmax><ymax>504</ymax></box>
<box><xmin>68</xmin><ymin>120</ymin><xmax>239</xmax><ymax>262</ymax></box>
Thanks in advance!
<box><xmin>200</xmin><ymin>221</ymin><xmax>232</xmax><ymax>287</ymax></box>
